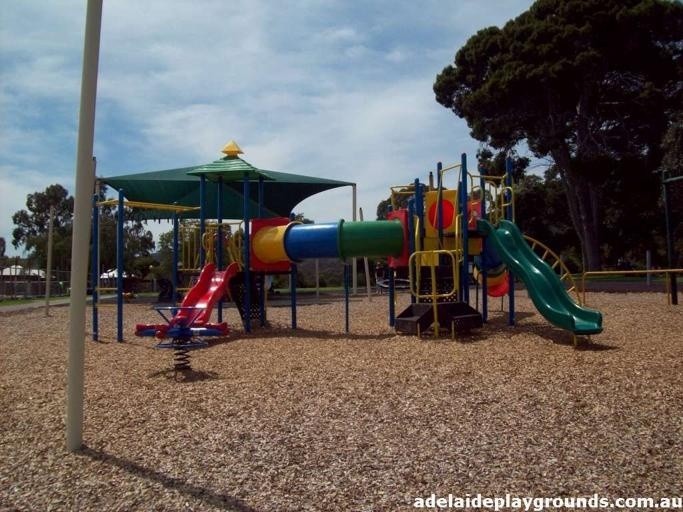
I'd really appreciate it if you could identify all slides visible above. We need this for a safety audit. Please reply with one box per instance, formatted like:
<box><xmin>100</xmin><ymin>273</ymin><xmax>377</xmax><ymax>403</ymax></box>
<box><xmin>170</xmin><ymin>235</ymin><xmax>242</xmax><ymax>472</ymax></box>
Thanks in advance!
<box><xmin>172</xmin><ymin>261</ymin><xmax>241</xmax><ymax>323</ymax></box>
<box><xmin>476</xmin><ymin>216</ymin><xmax>603</xmax><ymax>336</ymax></box>
